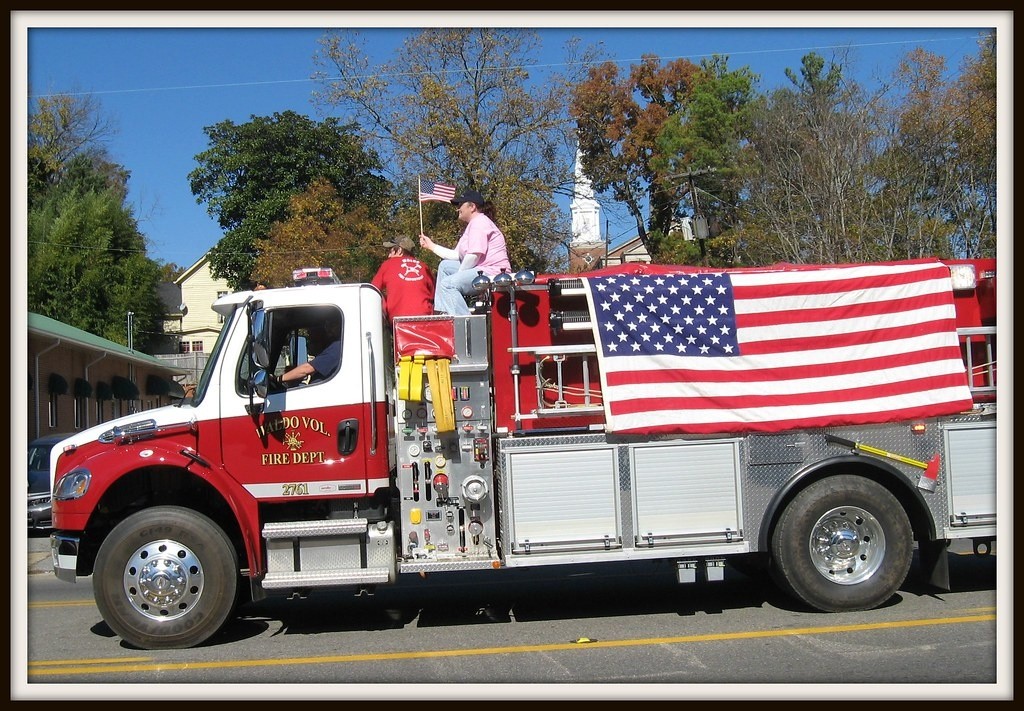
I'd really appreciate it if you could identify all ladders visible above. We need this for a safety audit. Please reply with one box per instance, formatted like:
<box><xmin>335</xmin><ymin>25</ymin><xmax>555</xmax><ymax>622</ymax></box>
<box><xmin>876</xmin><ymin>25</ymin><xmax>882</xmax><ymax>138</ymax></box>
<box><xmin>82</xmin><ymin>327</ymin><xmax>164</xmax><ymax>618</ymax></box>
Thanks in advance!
<box><xmin>508</xmin><ymin>326</ymin><xmax>997</xmax><ymax>419</ymax></box>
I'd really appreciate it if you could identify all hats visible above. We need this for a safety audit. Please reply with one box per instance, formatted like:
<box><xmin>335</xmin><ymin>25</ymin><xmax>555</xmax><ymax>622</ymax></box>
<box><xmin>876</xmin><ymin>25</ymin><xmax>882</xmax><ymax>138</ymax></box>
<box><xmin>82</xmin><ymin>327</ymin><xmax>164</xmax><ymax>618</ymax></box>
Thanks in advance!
<box><xmin>383</xmin><ymin>236</ymin><xmax>415</xmax><ymax>252</ymax></box>
<box><xmin>450</xmin><ymin>191</ymin><xmax>484</xmax><ymax>206</ymax></box>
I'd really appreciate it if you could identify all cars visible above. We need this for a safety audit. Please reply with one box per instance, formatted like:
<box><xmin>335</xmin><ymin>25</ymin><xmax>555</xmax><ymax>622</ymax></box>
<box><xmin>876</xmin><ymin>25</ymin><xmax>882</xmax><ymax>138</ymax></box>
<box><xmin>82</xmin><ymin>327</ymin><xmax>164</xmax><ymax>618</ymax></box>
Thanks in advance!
<box><xmin>27</xmin><ymin>434</ymin><xmax>73</xmax><ymax>529</ymax></box>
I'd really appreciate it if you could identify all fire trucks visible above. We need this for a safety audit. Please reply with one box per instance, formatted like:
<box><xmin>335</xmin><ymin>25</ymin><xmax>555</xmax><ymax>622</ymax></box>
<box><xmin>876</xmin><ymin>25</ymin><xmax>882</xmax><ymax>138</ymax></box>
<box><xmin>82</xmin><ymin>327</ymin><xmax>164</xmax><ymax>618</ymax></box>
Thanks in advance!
<box><xmin>47</xmin><ymin>256</ymin><xmax>998</xmax><ymax>649</ymax></box>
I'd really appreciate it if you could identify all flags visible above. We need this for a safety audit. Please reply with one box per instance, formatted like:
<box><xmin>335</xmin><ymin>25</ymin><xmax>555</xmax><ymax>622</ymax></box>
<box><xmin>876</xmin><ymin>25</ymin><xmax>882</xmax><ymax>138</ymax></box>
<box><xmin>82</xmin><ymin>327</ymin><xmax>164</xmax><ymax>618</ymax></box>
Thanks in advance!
<box><xmin>580</xmin><ymin>255</ymin><xmax>973</xmax><ymax>435</ymax></box>
<box><xmin>418</xmin><ymin>179</ymin><xmax>456</xmax><ymax>202</ymax></box>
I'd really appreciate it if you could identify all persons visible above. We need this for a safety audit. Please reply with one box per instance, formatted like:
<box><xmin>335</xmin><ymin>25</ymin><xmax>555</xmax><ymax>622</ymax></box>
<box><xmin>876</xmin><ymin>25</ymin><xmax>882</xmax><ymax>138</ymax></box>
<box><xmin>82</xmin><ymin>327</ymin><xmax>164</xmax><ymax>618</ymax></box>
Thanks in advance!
<box><xmin>269</xmin><ymin>312</ymin><xmax>341</xmax><ymax>384</ymax></box>
<box><xmin>418</xmin><ymin>190</ymin><xmax>511</xmax><ymax>315</ymax></box>
<box><xmin>371</xmin><ymin>234</ymin><xmax>435</xmax><ymax>330</ymax></box>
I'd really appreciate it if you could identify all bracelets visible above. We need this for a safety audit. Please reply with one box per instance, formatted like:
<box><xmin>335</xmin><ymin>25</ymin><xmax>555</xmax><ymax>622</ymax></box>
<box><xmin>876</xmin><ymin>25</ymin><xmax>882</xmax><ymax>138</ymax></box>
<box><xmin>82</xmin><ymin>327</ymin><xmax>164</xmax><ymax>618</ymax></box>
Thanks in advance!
<box><xmin>279</xmin><ymin>375</ymin><xmax>283</xmax><ymax>383</ymax></box>
